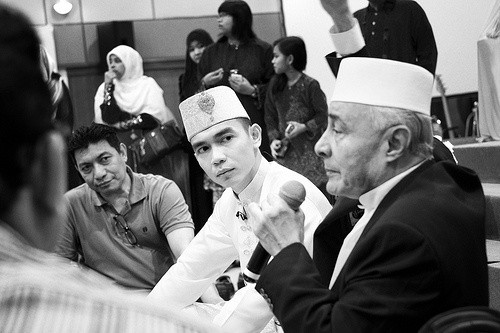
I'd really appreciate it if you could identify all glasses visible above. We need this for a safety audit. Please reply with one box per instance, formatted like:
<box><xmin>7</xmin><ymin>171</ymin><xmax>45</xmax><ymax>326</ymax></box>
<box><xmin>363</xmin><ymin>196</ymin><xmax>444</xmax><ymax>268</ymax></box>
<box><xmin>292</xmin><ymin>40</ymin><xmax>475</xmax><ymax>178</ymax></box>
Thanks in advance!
<box><xmin>114</xmin><ymin>215</ymin><xmax>140</xmax><ymax>248</ymax></box>
<box><xmin>216</xmin><ymin>13</ymin><xmax>229</xmax><ymax>18</ymax></box>
<box><xmin>39</xmin><ymin>68</ymin><xmax>74</xmax><ymax>163</ymax></box>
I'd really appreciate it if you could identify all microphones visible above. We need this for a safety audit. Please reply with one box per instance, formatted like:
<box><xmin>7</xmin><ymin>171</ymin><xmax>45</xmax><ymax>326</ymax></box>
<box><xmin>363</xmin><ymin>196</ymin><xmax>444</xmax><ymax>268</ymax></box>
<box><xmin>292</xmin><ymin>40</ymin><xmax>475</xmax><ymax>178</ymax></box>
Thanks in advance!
<box><xmin>243</xmin><ymin>180</ymin><xmax>306</xmax><ymax>284</ymax></box>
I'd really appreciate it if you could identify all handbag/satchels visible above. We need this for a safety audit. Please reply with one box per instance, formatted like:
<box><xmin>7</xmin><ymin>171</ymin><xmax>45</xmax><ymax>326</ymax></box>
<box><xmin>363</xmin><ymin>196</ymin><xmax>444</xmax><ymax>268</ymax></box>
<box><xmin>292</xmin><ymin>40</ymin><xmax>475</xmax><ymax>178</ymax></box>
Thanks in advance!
<box><xmin>120</xmin><ymin>114</ymin><xmax>183</xmax><ymax>173</ymax></box>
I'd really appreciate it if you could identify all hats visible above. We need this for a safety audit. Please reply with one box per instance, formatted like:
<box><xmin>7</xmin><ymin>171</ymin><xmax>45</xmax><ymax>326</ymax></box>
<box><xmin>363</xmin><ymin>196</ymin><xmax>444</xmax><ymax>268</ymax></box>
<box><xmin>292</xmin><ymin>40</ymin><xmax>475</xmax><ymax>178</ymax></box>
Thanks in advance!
<box><xmin>331</xmin><ymin>54</ymin><xmax>435</xmax><ymax>114</ymax></box>
<box><xmin>178</xmin><ymin>85</ymin><xmax>251</xmax><ymax>140</ymax></box>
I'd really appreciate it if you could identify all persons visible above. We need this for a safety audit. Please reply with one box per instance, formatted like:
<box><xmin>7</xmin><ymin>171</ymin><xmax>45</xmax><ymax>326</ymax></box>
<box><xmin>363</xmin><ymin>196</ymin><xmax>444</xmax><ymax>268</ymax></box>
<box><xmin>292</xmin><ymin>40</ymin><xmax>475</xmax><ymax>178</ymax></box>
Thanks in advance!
<box><xmin>353</xmin><ymin>0</ymin><xmax>438</xmax><ymax>76</ymax></box>
<box><xmin>143</xmin><ymin>85</ymin><xmax>334</xmax><ymax>333</ymax></box>
<box><xmin>197</xmin><ymin>0</ymin><xmax>277</xmax><ymax>161</ymax></box>
<box><xmin>264</xmin><ymin>35</ymin><xmax>334</xmax><ymax>205</ymax></box>
<box><xmin>0</xmin><ymin>2</ymin><xmax>219</xmax><ymax>333</ymax></box>
<box><xmin>93</xmin><ymin>46</ymin><xmax>179</xmax><ymax>181</ymax></box>
<box><xmin>38</xmin><ymin>46</ymin><xmax>74</xmax><ymax>142</ymax></box>
<box><xmin>54</xmin><ymin>122</ymin><xmax>227</xmax><ymax>305</ymax></box>
<box><xmin>179</xmin><ymin>28</ymin><xmax>215</xmax><ymax>236</ymax></box>
<box><xmin>246</xmin><ymin>0</ymin><xmax>491</xmax><ymax>333</ymax></box>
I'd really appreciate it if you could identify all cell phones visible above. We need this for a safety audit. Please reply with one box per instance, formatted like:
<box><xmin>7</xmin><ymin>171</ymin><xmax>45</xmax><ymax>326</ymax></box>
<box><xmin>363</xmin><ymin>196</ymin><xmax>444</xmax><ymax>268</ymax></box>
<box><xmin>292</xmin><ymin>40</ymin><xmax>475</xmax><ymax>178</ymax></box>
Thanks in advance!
<box><xmin>286</xmin><ymin>124</ymin><xmax>294</xmax><ymax>134</ymax></box>
<box><xmin>231</xmin><ymin>74</ymin><xmax>242</xmax><ymax>83</ymax></box>
